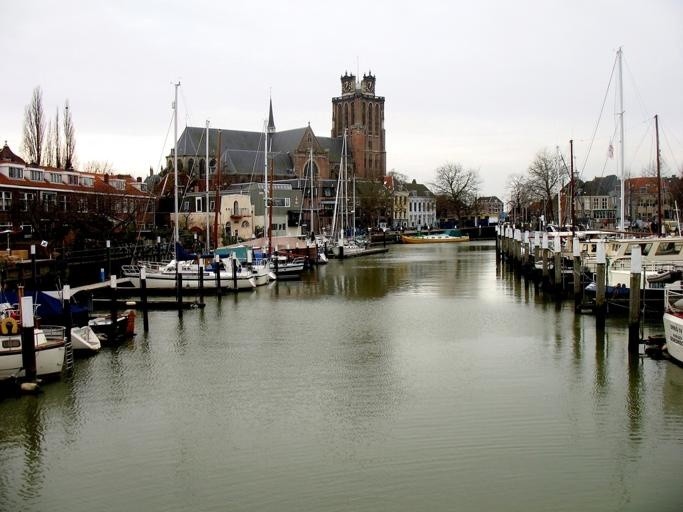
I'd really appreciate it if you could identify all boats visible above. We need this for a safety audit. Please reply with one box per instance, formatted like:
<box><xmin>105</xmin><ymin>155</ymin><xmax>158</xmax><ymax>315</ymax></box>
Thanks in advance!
<box><xmin>0</xmin><ymin>281</ymin><xmax>67</xmax><ymax>381</ymax></box>
<box><xmin>660</xmin><ymin>280</ymin><xmax>683</xmax><ymax>366</ymax></box>
<box><xmin>34</xmin><ymin>287</ymin><xmax>105</xmax><ymax>352</ymax></box>
<box><xmin>395</xmin><ymin>230</ymin><xmax>472</xmax><ymax>244</ymax></box>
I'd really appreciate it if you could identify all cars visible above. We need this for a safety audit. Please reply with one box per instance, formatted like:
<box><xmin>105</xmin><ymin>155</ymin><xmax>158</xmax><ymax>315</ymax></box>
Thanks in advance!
<box><xmin>390</xmin><ymin>226</ymin><xmax>407</xmax><ymax>231</ymax></box>
<box><xmin>420</xmin><ymin>226</ymin><xmax>430</xmax><ymax>230</ymax></box>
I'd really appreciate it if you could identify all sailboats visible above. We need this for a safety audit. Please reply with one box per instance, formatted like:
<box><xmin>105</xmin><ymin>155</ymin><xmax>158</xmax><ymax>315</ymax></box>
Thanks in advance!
<box><xmin>519</xmin><ymin>47</ymin><xmax>682</xmax><ymax>298</ymax></box>
<box><xmin>199</xmin><ymin>96</ymin><xmax>367</xmax><ymax>275</ymax></box>
<box><xmin>121</xmin><ymin>82</ymin><xmax>259</xmax><ymax>292</ymax></box>
